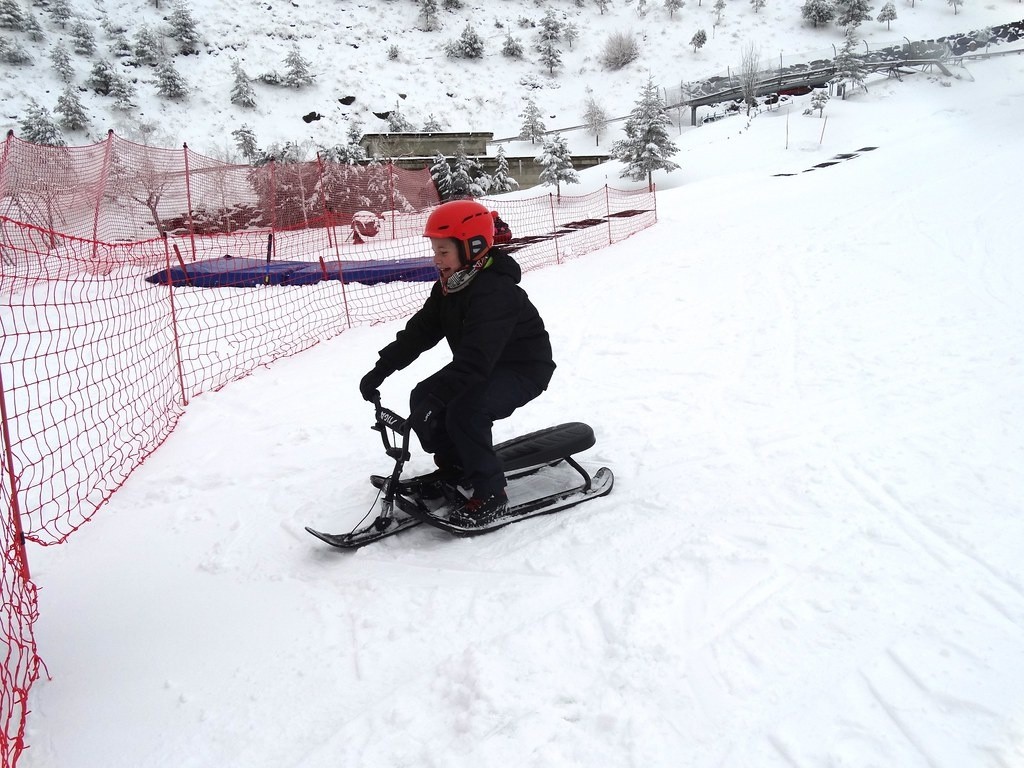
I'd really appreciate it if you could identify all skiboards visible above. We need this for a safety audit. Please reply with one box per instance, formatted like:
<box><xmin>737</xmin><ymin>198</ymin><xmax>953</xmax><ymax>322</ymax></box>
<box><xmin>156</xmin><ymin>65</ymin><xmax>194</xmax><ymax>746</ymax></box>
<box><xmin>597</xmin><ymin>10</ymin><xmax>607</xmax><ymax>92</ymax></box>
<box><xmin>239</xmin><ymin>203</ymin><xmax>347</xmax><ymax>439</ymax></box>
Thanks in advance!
<box><xmin>303</xmin><ymin>464</ymin><xmax>617</xmax><ymax>551</ymax></box>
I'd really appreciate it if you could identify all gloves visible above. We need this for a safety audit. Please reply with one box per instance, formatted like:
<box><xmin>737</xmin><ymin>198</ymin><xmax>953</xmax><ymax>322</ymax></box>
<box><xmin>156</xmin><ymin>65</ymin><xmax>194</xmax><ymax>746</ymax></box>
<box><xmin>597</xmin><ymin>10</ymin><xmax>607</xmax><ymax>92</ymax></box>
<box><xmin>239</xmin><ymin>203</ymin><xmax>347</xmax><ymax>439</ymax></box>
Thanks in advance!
<box><xmin>410</xmin><ymin>405</ymin><xmax>435</xmax><ymax>433</ymax></box>
<box><xmin>359</xmin><ymin>368</ymin><xmax>387</xmax><ymax>402</ymax></box>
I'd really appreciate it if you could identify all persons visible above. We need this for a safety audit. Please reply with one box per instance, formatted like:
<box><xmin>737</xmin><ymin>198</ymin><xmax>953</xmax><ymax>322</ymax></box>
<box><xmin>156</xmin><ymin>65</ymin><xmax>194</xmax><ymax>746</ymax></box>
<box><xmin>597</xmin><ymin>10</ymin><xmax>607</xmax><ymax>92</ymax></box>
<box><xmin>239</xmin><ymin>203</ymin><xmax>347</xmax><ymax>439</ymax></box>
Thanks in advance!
<box><xmin>491</xmin><ymin>211</ymin><xmax>508</xmax><ymax>235</ymax></box>
<box><xmin>359</xmin><ymin>199</ymin><xmax>557</xmax><ymax>529</ymax></box>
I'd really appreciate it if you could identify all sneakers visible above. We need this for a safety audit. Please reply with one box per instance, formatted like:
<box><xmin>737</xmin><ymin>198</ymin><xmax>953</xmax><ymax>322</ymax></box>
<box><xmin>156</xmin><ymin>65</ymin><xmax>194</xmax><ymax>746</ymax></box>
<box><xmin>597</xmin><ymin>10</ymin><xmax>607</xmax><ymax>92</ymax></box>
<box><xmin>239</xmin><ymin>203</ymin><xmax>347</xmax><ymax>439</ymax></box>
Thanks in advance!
<box><xmin>448</xmin><ymin>486</ymin><xmax>509</xmax><ymax>527</ymax></box>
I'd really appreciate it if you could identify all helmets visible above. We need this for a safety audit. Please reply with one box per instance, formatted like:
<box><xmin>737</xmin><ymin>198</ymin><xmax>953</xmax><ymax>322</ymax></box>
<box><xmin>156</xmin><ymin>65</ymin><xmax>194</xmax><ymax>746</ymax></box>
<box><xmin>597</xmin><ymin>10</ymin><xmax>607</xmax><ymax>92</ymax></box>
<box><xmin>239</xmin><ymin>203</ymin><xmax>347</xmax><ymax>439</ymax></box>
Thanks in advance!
<box><xmin>423</xmin><ymin>199</ymin><xmax>495</xmax><ymax>265</ymax></box>
